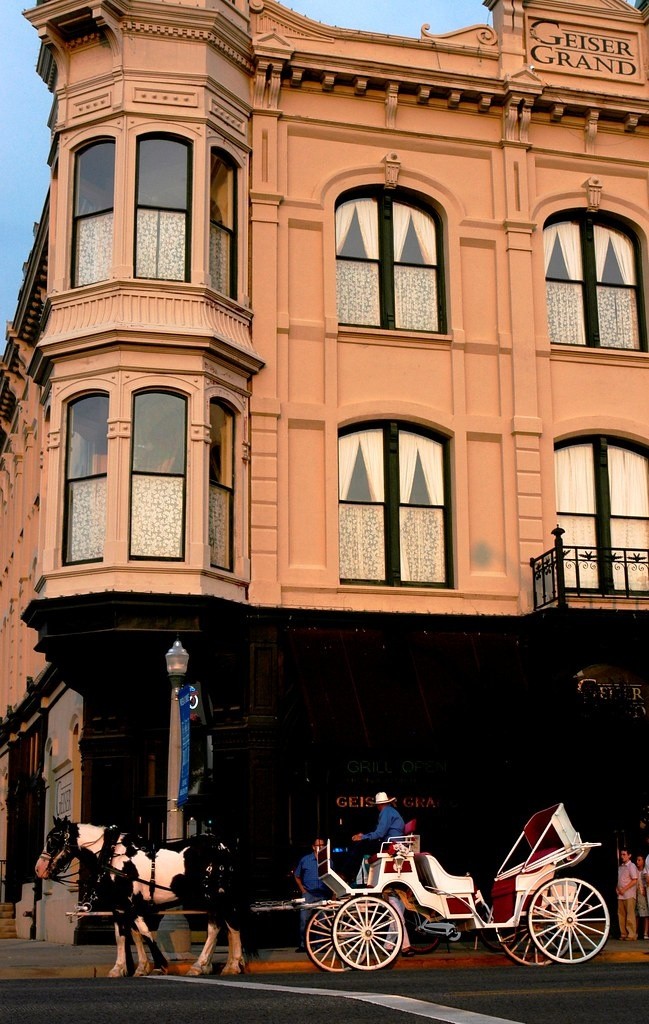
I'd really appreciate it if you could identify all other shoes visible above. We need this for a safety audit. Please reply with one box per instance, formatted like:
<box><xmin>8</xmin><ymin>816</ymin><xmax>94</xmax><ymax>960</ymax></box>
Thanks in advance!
<box><xmin>295</xmin><ymin>946</ymin><xmax>306</xmax><ymax>953</ymax></box>
<box><xmin>644</xmin><ymin>935</ymin><xmax>649</xmax><ymax>939</ymax></box>
<box><xmin>315</xmin><ymin>947</ymin><xmax>328</xmax><ymax>953</ymax></box>
<box><xmin>619</xmin><ymin>937</ymin><xmax>637</xmax><ymax>941</ymax></box>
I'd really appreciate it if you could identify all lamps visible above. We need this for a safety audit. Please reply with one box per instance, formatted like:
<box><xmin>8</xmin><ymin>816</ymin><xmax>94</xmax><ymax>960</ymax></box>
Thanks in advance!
<box><xmin>165</xmin><ymin>631</ymin><xmax>189</xmax><ymax>696</ymax></box>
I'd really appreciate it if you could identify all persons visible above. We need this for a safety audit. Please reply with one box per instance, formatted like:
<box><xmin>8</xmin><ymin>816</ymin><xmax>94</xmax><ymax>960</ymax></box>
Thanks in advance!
<box><xmin>381</xmin><ymin>893</ymin><xmax>414</xmax><ymax>957</ymax></box>
<box><xmin>294</xmin><ymin>837</ymin><xmax>335</xmax><ymax>952</ymax></box>
<box><xmin>616</xmin><ymin>847</ymin><xmax>649</xmax><ymax>940</ymax></box>
<box><xmin>351</xmin><ymin>791</ymin><xmax>405</xmax><ymax>850</ymax></box>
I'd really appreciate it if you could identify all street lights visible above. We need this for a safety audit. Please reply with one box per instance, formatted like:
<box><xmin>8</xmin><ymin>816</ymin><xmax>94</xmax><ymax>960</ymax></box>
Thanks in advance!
<box><xmin>155</xmin><ymin>637</ymin><xmax>193</xmax><ymax>954</ymax></box>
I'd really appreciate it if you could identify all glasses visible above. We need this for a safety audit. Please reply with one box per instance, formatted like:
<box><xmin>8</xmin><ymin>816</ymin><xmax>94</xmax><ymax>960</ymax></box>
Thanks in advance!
<box><xmin>314</xmin><ymin>844</ymin><xmax>325</xmax><ymax>846</ymax></box>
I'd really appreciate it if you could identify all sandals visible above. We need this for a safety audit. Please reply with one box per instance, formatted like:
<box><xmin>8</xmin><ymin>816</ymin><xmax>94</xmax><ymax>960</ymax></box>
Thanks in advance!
<box><xmin>382</xmin><ymin>950</ymin><xmax>393</xmax><ymax>956</ymax></box>
<box><xmin>402</xmin><ymin>949</ymin><xmax>416</xmax><ymax>957</ymax></box>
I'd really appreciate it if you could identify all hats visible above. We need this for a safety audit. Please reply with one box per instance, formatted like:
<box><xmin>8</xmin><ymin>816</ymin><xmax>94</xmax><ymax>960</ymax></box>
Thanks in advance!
<box><xmin>373</xmin><ymin>792</ymin><xmax>395</xmax><ymax>804</ymax></box>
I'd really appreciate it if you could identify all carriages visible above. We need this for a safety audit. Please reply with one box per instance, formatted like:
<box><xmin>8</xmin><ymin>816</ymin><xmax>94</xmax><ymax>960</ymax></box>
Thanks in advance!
<box><xmin>34</xmin><ymin>802</ymin><xmax>613</xmax><ymax>980</ymax></box>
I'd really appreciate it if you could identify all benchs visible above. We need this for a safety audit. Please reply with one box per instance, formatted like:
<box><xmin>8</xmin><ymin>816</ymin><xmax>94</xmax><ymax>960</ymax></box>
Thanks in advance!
<box><xmin>491</xmin><ymin>847</ymin><xmax>556</xmax><ymax>897</ymax></box>
<box><xmin>366</xmin><ymin>818</ymin><xmax>415</xmax><ymax>865</ymax></box>
<box><xmin>415</xmin><ymin>852</ymin><xmax>474</xmax><ymax>894</ymax></box>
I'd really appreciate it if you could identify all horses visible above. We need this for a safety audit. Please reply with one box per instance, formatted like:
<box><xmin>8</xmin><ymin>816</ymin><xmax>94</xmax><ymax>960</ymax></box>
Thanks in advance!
<box><xmin>35</xmin><ymin>815</ymin><xmax>261</xmax><ymax>976</ymax></box>
